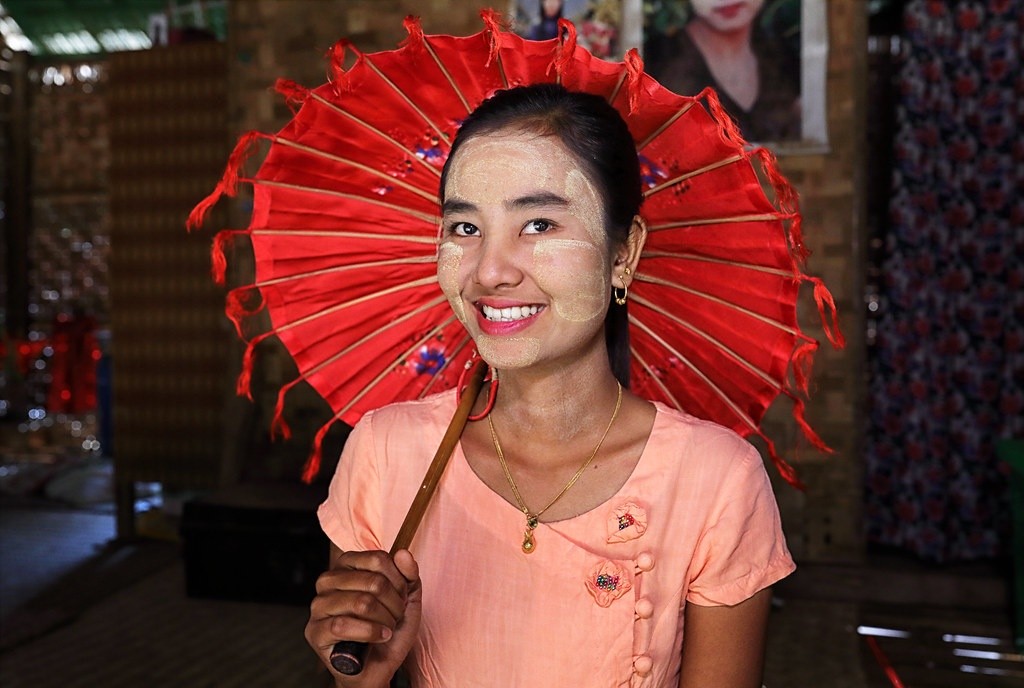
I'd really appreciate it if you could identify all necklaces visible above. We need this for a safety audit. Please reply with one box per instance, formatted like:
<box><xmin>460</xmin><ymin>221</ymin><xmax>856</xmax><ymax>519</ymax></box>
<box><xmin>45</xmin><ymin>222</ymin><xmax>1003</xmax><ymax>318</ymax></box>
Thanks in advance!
<box><xmin>484</xmin><ymin>378</ymin><xmax>623</xmax><ymax>554</ymax></box>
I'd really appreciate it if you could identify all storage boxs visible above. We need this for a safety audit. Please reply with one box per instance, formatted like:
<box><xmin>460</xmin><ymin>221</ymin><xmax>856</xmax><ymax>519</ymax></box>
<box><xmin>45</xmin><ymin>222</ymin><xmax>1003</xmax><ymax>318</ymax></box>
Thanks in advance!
<box><xmin>175</xmin><ymin>494</ymin><xmax>330</xmax><ymax>608</ymax></box>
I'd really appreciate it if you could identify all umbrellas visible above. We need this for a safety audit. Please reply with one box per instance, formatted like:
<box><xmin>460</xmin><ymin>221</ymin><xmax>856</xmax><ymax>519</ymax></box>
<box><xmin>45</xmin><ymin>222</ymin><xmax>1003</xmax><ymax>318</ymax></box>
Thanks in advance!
<box><xmin>184</xmin><ymin>6</ymin><xmax>847</xmax><ymax>676</ymax></box>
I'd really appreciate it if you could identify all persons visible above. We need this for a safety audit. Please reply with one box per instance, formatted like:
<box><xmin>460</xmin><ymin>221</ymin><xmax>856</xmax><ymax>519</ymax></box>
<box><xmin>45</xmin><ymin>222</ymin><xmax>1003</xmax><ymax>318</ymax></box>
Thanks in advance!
<box><xmin>304</xmin><ymin>83</ymin><xmax>796</xmax><ymax>688</ymax></box>
<box><xmin>530</xmin><ymin>0</ymin><xmax>563</xmax><ymax>41</ymax></box>
<box><xmin>645</xmin><ymin>0</ymin><xmax>801</xmax><ymax>145</ymax></box>
